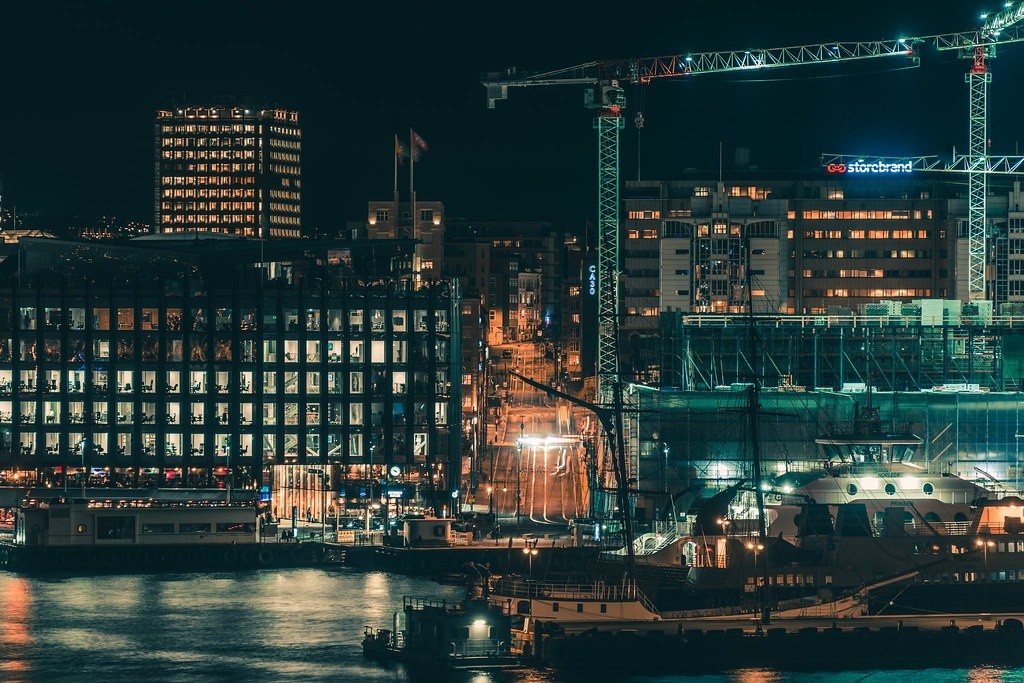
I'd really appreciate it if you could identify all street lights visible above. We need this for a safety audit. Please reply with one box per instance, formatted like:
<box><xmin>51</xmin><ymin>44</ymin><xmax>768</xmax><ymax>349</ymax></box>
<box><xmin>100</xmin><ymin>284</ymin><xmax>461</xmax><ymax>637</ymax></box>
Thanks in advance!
<box><xmin>226</xmin><ymin>433</ymin><xmax>232</xmax><ymax>504</ymax></box>
<box><xmin>81</xmin><ymin>437</ymin><xmax>87</xmax><ymax>497</ymax></box>
<box><xmin>369</xmin><ymin>445</ymin><xmax>376</xmax><ymax>503</ymax></box>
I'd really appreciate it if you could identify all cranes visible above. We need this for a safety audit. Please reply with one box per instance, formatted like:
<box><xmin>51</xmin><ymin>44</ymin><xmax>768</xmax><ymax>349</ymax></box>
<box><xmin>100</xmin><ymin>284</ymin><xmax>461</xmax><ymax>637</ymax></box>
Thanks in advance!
<box><xmin>819</xmin><ymin>0</ymin><xmax>1024</xmax><ymax>359</ymax></box>
<box><xmin>479</xmin><ymin>33</ymin><xmax>925</xmax><ymax>524</ymax></box>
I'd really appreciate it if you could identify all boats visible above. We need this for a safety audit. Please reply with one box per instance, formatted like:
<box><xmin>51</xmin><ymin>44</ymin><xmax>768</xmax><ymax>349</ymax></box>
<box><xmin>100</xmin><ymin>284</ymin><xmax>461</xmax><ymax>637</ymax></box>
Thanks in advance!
<box><xmin>461</xmin><ymin>218</ymin><xmax>1024</xmax><ymax>673</ymax></box>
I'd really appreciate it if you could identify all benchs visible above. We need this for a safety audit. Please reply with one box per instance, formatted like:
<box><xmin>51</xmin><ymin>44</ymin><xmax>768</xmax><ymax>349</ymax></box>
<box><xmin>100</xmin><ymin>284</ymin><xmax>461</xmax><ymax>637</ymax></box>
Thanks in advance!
<box><xmin>279</xmin><ymin>537</ymin><xmax>300</xmax><ymax>542</ymax></box>
